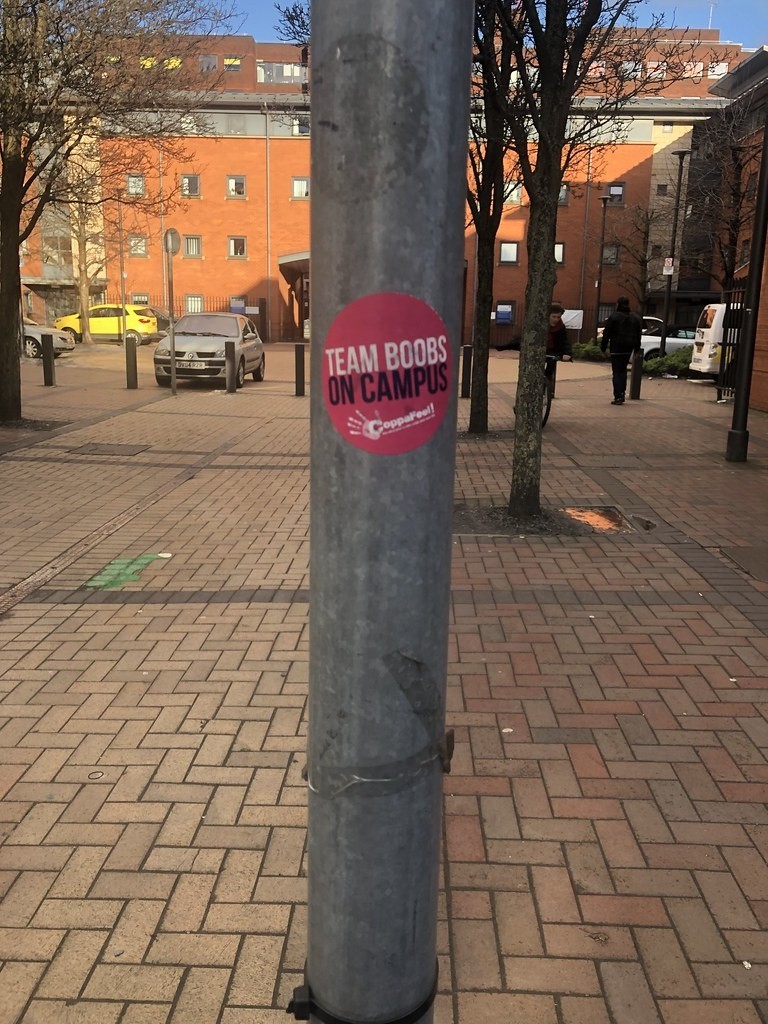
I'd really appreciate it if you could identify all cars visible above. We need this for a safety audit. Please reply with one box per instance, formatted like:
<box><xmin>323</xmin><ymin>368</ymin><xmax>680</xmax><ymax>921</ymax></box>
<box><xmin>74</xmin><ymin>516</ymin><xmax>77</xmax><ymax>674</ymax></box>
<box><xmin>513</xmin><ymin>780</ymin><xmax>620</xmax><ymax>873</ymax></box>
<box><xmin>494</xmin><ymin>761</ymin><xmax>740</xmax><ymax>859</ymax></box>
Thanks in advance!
<box><xmin>18</xmin><ymin>314</ymin><xmax>76</xmax><ymax>359</ymax></box>
<box><xmin>53</xmin><ymin>304</ymin><xmax>179</xmax><ymax>346</ymax></box>
<box><xmin>597</xmin><ymin>316</ymin><xmax>695</xmax><ymax>362</ymax></box>
<box><xmin>153</xmin><ymin>312</ymin><xmax>266</xmax><ymax>387</ymax></box>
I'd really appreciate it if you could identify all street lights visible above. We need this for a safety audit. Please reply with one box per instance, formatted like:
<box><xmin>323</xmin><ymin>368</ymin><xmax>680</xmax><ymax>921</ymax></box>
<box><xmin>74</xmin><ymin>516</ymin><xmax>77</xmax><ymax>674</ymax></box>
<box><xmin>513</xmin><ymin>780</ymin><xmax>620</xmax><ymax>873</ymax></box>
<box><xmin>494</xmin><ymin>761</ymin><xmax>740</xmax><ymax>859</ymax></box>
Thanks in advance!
<box><xmin>659</xmin><ymin>149</ymin><xmax>693</xmax><ymax>359</ymax></box>
<box><xmin>594</xmin><ymin>195</ymin><xmax>612</xmax><ymax>345</ymax></box>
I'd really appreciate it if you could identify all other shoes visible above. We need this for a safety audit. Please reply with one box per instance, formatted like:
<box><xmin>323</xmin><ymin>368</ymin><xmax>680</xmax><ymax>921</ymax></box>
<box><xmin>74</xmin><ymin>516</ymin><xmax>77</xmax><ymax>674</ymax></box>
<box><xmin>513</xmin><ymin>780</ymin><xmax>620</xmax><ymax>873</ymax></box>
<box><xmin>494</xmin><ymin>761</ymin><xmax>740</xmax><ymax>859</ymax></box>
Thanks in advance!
<box><xmin>622</xmin><ymin>396</ymin><xmax>625</xmax><ymax>402</ymax></box>
<box><xmin>611</xmin><ymin>399</ymin><xmax>624</xmax><ymax>405</ymax></box>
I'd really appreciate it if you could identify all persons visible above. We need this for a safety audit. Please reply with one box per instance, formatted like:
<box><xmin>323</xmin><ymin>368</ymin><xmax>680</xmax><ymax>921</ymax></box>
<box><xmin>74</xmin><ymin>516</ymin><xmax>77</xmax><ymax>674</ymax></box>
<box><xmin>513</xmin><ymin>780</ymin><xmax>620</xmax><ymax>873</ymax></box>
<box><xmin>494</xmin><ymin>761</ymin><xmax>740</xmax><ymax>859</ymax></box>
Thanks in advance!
<box><xmin>600</xmin><ymin>297</ymin><xmax>642</xmax><ymax>406</ymax></box>
<box><xmin>546</xmin><ymin>305</ymin><xmax>573</xmax><ymax>378</ymax></box>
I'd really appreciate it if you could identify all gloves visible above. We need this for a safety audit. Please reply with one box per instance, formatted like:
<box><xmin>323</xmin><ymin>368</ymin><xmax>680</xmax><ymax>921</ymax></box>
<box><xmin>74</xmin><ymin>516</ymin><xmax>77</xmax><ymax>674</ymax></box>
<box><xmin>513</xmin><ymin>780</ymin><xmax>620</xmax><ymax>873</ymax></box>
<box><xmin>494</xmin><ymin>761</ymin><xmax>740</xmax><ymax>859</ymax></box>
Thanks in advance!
<box><xmin>562</xmin><ymin>354</ymin><xmax>571</xmax><ymax>361</ymax></box>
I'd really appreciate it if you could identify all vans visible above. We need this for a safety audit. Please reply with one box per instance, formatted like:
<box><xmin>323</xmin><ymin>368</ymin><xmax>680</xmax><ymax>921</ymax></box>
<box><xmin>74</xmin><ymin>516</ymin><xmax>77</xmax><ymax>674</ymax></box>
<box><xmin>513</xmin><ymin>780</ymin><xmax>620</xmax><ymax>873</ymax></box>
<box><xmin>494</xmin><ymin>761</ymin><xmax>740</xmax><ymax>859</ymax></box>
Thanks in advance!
<box><xmin>688</xmin><ymin>303</ymin><xmax>752</xmax><ymax>388</ymax></box>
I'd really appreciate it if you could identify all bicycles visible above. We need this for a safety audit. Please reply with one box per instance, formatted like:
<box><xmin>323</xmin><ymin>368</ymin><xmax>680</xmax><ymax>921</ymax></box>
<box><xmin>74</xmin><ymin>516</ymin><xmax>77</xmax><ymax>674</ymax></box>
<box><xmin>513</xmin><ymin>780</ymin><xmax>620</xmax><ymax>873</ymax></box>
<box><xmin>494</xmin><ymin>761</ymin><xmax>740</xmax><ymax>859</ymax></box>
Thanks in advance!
<box><xmin>540</xmin><ymin>354</ymin><xmax>573</xmax><ymax>428</ymax></box>
